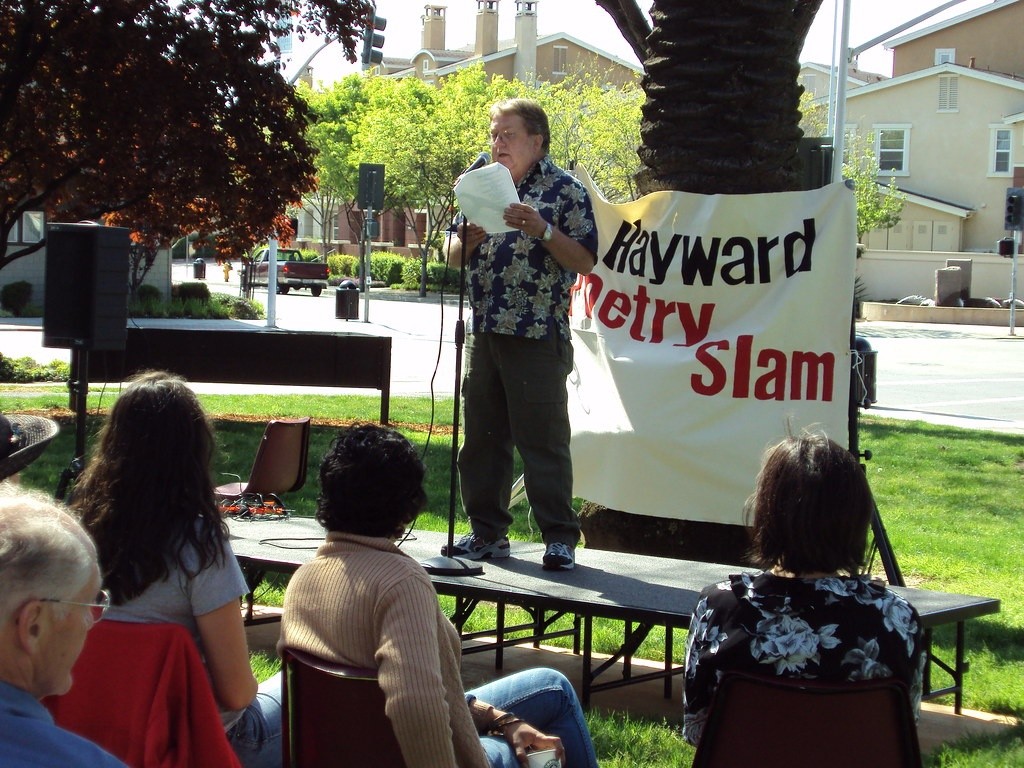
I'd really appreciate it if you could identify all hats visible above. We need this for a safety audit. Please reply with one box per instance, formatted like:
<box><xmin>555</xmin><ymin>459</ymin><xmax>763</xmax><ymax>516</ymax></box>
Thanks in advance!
<box><xmin>0</xmin><ymin>412</ymin><xmax>61</xmax><ymax>484</ymax></box>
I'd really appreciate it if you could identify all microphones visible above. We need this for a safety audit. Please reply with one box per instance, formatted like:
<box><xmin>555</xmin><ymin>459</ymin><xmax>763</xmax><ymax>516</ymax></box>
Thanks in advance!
<box><xmin>454</xmin><ymin>153</ymin><xmax>491</xmax><ymax>186</ymax></box>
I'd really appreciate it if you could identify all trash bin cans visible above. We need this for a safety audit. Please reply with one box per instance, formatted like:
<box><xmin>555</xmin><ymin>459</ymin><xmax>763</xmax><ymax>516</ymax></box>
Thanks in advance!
<box><xmin>335</xmin><ymin>279</ymin><xmax>360</xmax><ymax>319</ymax></box>
<box><xmin>193</xmin><ymin>258</ymin><xmax>206</xmax><ymax>278</ymax></box>
<box><xmin>852</xmin><ymin>337</ymin><xmax>878</xmax><ymax>405</ymax></box>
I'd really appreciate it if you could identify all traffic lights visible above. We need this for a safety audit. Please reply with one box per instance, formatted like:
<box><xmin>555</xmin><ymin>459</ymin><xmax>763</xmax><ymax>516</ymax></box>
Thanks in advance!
<box><xmin>361</xmin><ymin>6</ymin><xmax>386</xmax><ymax>65</ymax></box>
<box><xmin>998</xmin><ymin>237</ymin><xmax>1015</xmax><ymax>256</ymax></box>
<box><xmin>1004</xmin><ymin>188</ymin><xmax>1024</xmax><ymax>230</ymax></box>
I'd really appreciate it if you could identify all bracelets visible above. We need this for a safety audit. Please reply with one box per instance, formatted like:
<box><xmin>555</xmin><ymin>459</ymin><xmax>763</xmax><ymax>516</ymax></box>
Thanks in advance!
<box><xmin>487</xmin><ymin>712</ymin><xmax>527</xmax><ymax>736</ymax></box>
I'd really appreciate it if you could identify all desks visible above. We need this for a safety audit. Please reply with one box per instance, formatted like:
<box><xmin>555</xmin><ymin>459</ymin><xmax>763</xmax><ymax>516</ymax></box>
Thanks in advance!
<box><xmin>68</xmin><ymin>318</ymin><xmax>394</xmax><ymax>471</ymax></box>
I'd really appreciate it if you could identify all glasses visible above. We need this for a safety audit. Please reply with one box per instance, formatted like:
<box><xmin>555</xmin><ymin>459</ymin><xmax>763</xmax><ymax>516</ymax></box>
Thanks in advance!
<box><xmin>15</xmin><ymin>589</ymin><xmax>110</xmax><ymax>624</ymax></box>
<box><xmin>484</xmin><ymin>126</ymin><xmax>516</xmax><ymax>146</ymax></box>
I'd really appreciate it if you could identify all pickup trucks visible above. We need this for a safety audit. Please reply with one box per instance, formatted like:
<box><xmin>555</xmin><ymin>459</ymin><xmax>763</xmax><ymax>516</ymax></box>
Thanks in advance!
<box><xmin>240</xmin><ymin>248</ymin><xmax>331</xmax><ymax>297</ymax></box>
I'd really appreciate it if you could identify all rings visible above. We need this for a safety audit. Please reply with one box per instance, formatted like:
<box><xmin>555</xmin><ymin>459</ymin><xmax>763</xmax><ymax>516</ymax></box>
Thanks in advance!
<box><xmin>521</xmin><ymin>219</ymin><xmax>526</xmax><ymax>226</ymax></box>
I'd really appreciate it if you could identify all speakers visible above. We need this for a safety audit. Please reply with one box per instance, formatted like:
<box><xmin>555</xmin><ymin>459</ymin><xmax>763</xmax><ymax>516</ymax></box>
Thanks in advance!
<box><xmin>42</xmin><ymin>219</ymin><xmax>131</xmax><ymax>352</ymax></box>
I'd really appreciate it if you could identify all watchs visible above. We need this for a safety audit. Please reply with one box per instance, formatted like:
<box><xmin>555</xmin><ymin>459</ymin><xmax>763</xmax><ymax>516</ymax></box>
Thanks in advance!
<box><xmin>537</xmin><ymin>222</ymin><xmax>553</xmax><ymax>242</ymax></box>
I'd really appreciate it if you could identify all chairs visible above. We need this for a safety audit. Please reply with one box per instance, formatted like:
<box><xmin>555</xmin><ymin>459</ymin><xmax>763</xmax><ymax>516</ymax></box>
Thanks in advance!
<box><xmin>41</xmin><ymin>618</ymin><xmax>247</xmax><ymax>768</ymax></box>
<box><xmin>281</xmin><ymin>647</ymin><xmax>407</xmax><ymax>768</ymax></box>
<box><xmin>690</xmin><ymin>667</ymin><xmax>924</xmax><ymax>768</ymax></box>
<box><xmin>214</xmin><ymin>416</ymin><xmax>311</xmax><ymax>504</ymax></box>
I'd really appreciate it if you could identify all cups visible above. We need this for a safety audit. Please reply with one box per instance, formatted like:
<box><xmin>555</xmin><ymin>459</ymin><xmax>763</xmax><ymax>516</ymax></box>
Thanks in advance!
<box><xmin>526</xmin><ymin>748</ymin><xmax>561</xmax><ymax>768</ymax></box>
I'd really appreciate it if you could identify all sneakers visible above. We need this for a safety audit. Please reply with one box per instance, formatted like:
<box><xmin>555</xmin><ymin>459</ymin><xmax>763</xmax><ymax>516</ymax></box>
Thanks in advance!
<box><xmin>441</xmin><ymin>532</ymin><xmax>510</xmax><ymax>560</ymax></box>
<box><xmin>541</xmin><ymin>542</ymin><xmax>575</xmax><ymax>569</ymax></box>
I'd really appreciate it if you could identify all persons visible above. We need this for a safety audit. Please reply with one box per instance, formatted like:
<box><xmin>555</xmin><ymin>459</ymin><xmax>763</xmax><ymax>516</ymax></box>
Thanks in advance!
<box><xmin>0</xmin><ymin>479</ymin><xmax>130</xmax><ymax>768</ymax></box>
<box><xmin>70</xmin><ymin>371</ymin><xmax>282</xmax><ymax>768</ymax></box>
<box><xmin>440</xmin><ymin>97</ymin><xmax>599</xmax><ymax>572</ymax></box>
<box><xmin>682</xmin><ymin>432</ymin><xmax>927</xmax><ymax>749</ymax></box>
<box><xmin>0</xmin><ymin>411</ymin><xmax>60</xmax><ymax>486</ymax></box>
<box><xmin>275</xmin><ymin>420</ymin><xmax>600</xmax><ymax>768</ymax></box>
